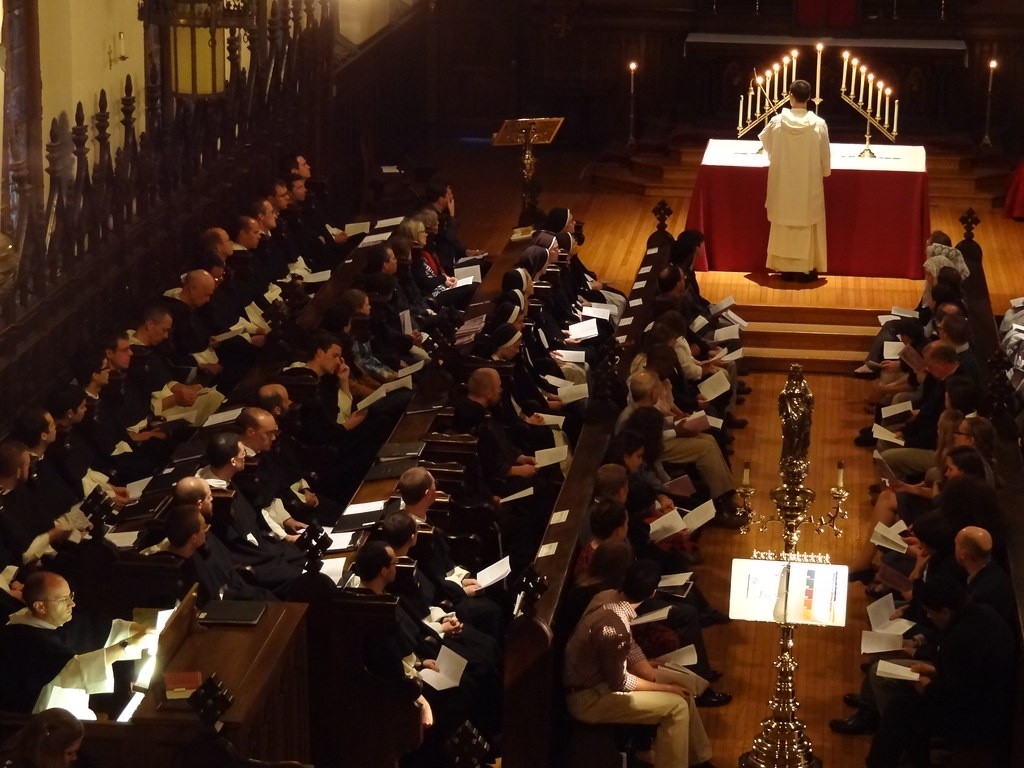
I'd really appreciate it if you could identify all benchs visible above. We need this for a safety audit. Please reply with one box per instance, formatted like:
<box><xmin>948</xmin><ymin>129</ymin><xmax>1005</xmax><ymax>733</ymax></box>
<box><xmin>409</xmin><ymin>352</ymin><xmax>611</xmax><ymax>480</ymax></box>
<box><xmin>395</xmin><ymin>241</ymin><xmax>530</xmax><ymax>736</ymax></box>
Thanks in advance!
<box><xmin>953</xmin><ymin>239</ymin><xmax>1024</xmax><ymax>643</ymax></box>
<box><xmin>112</xmin><ymin>216</ymin><xmax>675</xmax><ymax>767</ymax></box>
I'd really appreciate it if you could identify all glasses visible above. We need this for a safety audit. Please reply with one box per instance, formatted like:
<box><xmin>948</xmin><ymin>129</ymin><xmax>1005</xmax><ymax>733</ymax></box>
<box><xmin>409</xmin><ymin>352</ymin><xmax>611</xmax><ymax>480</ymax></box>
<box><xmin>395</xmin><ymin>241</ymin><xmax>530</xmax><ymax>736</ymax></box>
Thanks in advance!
<box><xmin>95</xmin><ymin>363</ymin><xmax>111</xmax><ymax>374</ymax></box>
<box><xmin>196</xmin><ymin>524</ymin><xmax>211</xmax><ymax>534</ymax></box>
<box><xmin>954</xmin><ymin>427</ymin><xmax>974</xmax><ymax>436</ymax></box>
<box><xmin>37</xmin><ymin>590</ymin><xmax>75</xmax><ymax>605</ymax></box>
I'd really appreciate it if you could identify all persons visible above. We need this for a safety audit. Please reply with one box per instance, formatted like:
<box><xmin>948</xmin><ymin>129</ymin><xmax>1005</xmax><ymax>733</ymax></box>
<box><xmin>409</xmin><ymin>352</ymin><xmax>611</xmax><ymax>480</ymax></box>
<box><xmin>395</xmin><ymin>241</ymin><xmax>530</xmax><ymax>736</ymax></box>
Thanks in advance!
<box><xmin>778</xmin><ymin>364</ymin><xmax>816</xmax><ymax>462</ymax></box>
<box><xmin>827</xmin><ymin>230</ymin><xmax>1024</xmax><ymax>768</ymax></box>
<box><xmin>757</xmin><ymin>80</ymin><xmax>831</xmax><ymax>283</ymax></box>
<box><xmin>563</xmin><ymin>232</ymin><xmax>751</xmax><ymax>768</ymax></box>
<box><xmin>1</xmin><ymin>152</ymin><xmax>622</xmax><ymax>768</ymax></box>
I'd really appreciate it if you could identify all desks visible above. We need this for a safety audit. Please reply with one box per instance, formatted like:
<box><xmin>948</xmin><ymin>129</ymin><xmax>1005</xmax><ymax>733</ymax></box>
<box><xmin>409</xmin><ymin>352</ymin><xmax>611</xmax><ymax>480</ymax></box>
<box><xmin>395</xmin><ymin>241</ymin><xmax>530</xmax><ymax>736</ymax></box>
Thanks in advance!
<box><xmin>685</xmin><ymin>139</ymin><xmax>930</xmax><ymax>280</ymax></box>
<box><xmin>130</xmin><ymin>599</ymin><xmax>308</xmax><ymax>768</ymax></box>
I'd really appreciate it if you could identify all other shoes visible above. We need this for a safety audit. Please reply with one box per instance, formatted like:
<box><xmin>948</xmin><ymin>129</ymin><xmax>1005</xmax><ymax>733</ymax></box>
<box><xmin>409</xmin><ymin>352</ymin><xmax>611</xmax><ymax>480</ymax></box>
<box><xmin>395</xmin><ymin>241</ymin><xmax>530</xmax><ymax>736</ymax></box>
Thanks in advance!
<box><xmin>865</xmin><ymin>582</ymin><xmax>891</xmax><ymax>597</ymax></box>
<box><xmin>854</xmin><ymin>426</ymin><xmax>881</xmax><ymax>446</ymax></box>
<box><xmin>700</xmin><ymin>669</ymin><xmax>724</xmax><ymax>681</ymax></box>
<box><xmin>723</xmin><ymin>434</ymin><xmax>736</xmax><ymax>441</ymax></box>
<box><xmin>710</xmin><ymin>499</ymin><xmax>751</xmax><ymax>528</ymax></box>
<box><xmin>702</xmin><ymin>607</ymin><xmax>731</xmax><ymax>629</ymax></box>
<box><xmin>865</xmin><ymin>399</ymin><xmax>878</xmax><ymax>413</ymax></box>
<box><xmin>842</xmin><ymin>693</ymin><xmax>868</xmax><ymax>707</ymax></box>
<box><xmin>735</xmin><ymin>385</ymin><xmax>752</xmax><ymax>394</ymax></box>
<box><xmin>849</xmin><ymin>566</ymin><xmax>882</xmax><ymax>583</ymax></box>
<box><xmin>725</xmin><ymin>413</ymin><xmax>749</xmax><ymax>429</ymax></box>
<box><xmin>694</xmin><ymin>688</ymin><xmax>731</xmax><ymax>706</ymax></box>
<box><xmin>829</xmin><ymin>715</ymin><xmax>874</xmax><ymax>733</ymax></box>
<box><xmin>735</xmin><ymin>365</ymin><xmax>749</xmax><ymax>376</ymax></box>
<box><xmin>843</xmin><ymin>364</ymin><xmax>877</xmax><ymax>380</ymax></box>
<box><xmin>869</xmin><ymin>482</ymin><xmax>884</xmax><ymax>493</ymax></box>
<box><xmin>859</xmin><ymin>661</ymin><xmax>873</xmax><ymax>672</ymax></box>
<box><xmin>678</xmin><ymin>546</ymin><xmax>702</xmax><ymax>563</ymax></box>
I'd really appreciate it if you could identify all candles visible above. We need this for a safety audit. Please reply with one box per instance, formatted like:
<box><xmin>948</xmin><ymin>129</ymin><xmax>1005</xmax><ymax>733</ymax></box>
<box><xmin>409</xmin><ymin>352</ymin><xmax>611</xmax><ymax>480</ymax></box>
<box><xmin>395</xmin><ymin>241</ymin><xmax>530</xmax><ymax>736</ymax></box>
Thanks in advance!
<box><xmin>747</xmin><ymin>79</ymin><xmax>755</xmax><ymax>121</ymax></box>
<box><xmin>893</xmin><ymin>100</ymin><xmax>899</xmax><ymax>134</ymax></box>
<box><xmin>837</xmin><ymin>459</ymin><xmax>844</xmax><ymax>488</ymax></box>
<box><xmin>629</xmin><ymin>62</ymin><xmax>637</xmax><ymax>94</ymax></box>
<box><xmin>791</xmin><ymin>49</ymin><xmax>798</xmax><ymax>83</ymax></box>
<box><xmin>868</xmin><ymin>74</ymin><xmax>874</xmax><ymax>111</ymax></box>
<box><xmin>765</xmin><ymin>70</ymin><xmax>772</xmax><ymax>107</ymax></box>
<box><xmin>988</xmin><ymin>59</ymin><xmax>998</xmax><ymax>92</ymax></box>
<box><xmin>877</xmin><ymin>81</ymin><xmax>883</xmax><ymax>118</ymax></box>
<box><xmin>782</xmin><ymin>56</ymin><xmax>790</xmax><ymax>93</ymax></box>
<box><xmin>738</xmin><ymin>95</ymin><xmax>744</xmax><ymax>128</ymax></box>
<box><xmin>119</xmin><ymin>32</ymin><xmax>124</xmax><ymax>57</ymax></box>
<box><xmin>885</xmin><ymin>88</ymin><xmax>891</xmax><ymax>126</ymax></box>
<box><xmin>850</xmin><ymin>58</ymin><xmax>859</xmax><ymax>96</ymax></box>
<box><xmin>772</xmin><ymin>63</ymin><xmax>780</xmax><ymax>100</ymax></box>
<box><xmin>842</xmin><ymin>51</ymin><xmax>850</xmax><ymax>89</ymax></box>
<box><xmin>743</xmin><ymin>460</ymin><xmax>750</xmax><ymax>485</ymax></box>
<box><xmin>756</xmin><ymin>76</ymin><xmax>763</xmax><ymax>114</ymax></box>
<box><xmin>815</xmin><ymin>43</ymin><xmax>823</xmax><ymax>99</ymax></box>
<box><xmin>859</xmin><ymin>66</ymin><xmax>866</xmax><ymax>103</ymax></box>
